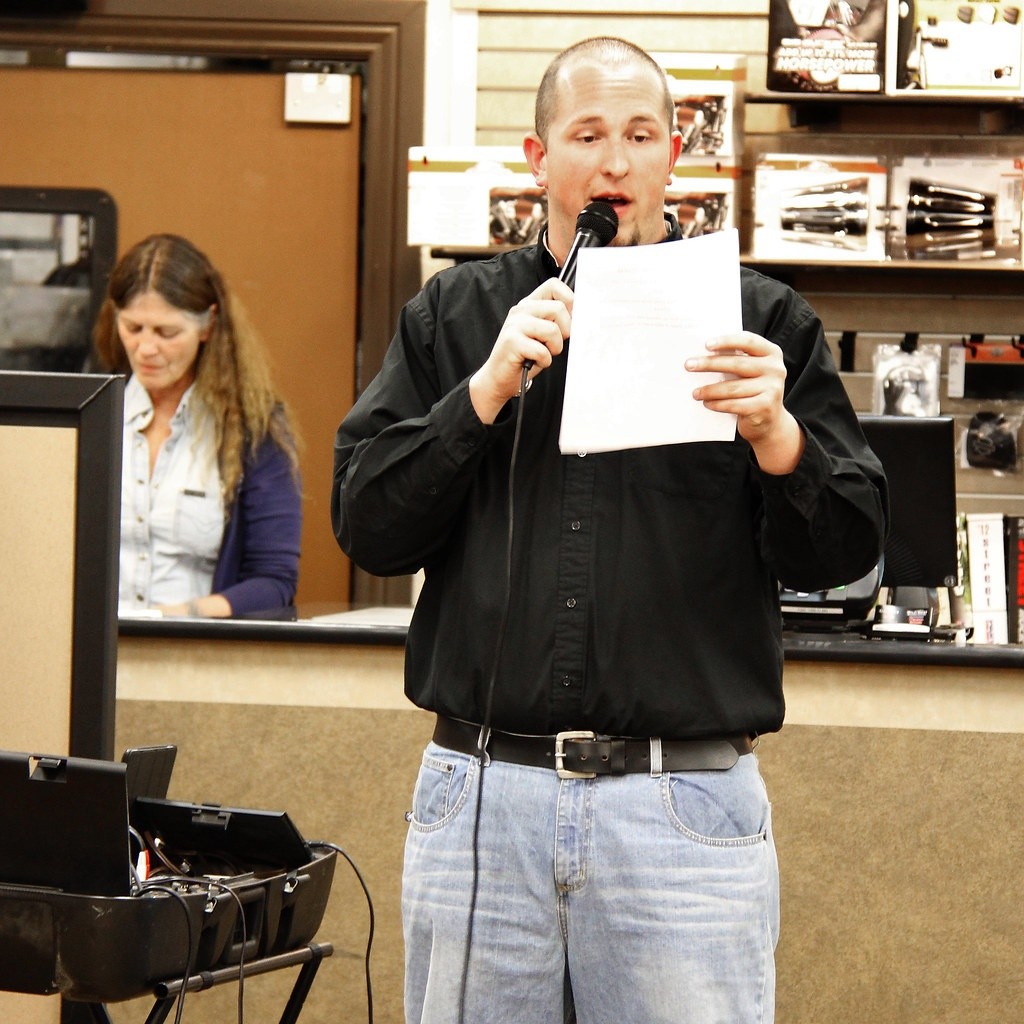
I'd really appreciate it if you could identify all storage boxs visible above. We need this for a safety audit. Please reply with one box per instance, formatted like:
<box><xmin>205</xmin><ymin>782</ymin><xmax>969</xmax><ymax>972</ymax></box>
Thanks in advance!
<box><xmin>1</xmin><ymin>842</ymin><xmax>340</xmax><ymax>1004</ymax></box>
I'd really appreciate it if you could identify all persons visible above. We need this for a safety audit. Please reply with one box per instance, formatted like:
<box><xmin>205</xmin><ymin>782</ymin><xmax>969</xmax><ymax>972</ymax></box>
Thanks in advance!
<box><xmin>78</xmin><ymin>233</ymin><xmax>300</xmax><ymax>629</ymax></box>
<box><xmin>326</xmin><ymin>33</ymin><xmax>892</xmax><ymax>1024</ymax></box>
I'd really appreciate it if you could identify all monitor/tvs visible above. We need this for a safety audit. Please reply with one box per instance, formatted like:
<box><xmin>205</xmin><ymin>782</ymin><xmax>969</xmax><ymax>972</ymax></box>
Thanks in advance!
<box><xmin>776</xmin><ymin>412</ymin><xmax>959</xmax><ymax>622</ymax></box>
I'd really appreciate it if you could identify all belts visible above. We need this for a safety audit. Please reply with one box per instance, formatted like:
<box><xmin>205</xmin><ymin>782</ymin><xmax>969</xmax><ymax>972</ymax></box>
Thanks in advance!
<box><xmin>430</xmin><ymin>717</ymin><xmax>758</xmax><ymax>781</ymax></box>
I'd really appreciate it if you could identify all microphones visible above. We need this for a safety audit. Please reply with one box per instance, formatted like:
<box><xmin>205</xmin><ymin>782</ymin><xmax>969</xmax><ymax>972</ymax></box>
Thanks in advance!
<box><xmin>522</xmin><ymin>200</ymin><xmax>620</xmax><ymax>370</ymax></box>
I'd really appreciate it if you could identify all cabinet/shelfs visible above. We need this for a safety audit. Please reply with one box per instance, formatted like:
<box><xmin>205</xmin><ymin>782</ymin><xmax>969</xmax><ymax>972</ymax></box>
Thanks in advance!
<box><xmin>729</xmin><ymin>87</ymin><xmax>1024</xmax><ymax>671</ymax></box>
